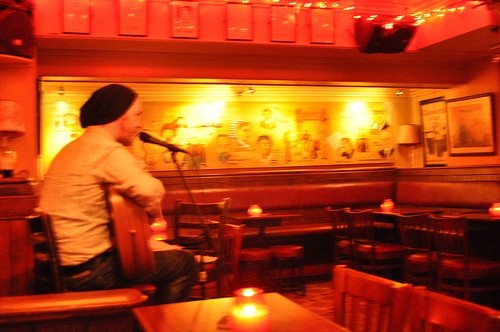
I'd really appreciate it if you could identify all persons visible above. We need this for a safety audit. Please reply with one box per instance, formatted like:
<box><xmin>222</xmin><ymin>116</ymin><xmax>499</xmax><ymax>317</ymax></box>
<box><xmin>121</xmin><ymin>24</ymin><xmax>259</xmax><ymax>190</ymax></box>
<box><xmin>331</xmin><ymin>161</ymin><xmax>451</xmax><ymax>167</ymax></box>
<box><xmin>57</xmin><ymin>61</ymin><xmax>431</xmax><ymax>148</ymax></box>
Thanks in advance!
<box><xmin>42</xmin><ymin>83</ymin><xmax>197</xmax><ymax>304</ymax></box>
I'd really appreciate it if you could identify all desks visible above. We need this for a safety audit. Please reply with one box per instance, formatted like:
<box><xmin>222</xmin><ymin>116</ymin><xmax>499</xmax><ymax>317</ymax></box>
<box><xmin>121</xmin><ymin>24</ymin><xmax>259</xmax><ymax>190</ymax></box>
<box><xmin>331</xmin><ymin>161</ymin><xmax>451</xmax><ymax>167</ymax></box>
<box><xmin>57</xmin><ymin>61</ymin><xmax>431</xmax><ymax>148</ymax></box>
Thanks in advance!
<box><xmin>448</xmin><ymin>214</ymin><xmax>500</xmax><ymax>258</ymax></box>
<box><xmin>226</xmin><ymin>210</ymin><xmax>302</xmax><ymax>248</ymax></box>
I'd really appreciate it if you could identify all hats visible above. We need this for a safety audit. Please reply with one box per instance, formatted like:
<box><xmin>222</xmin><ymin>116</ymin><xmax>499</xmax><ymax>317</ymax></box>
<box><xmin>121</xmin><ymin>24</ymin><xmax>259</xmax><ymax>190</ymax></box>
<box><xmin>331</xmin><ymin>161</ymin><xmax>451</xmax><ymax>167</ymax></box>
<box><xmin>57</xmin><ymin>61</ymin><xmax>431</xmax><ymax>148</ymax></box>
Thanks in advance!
<box><xmin>79</xmin><ymin>84</ymin><xmax>138</xmax><ymax>128</ymax></box>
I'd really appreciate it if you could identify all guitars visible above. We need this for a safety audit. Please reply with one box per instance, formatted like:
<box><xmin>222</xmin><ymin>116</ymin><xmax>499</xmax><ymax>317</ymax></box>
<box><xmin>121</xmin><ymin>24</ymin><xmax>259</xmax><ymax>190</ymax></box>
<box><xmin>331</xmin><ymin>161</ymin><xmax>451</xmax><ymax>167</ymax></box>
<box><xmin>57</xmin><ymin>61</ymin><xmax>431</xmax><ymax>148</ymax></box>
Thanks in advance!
<box><xmin>107</xmin><ymin>183</ymin><xmax>157</xmax><ymax>278</ymax></box>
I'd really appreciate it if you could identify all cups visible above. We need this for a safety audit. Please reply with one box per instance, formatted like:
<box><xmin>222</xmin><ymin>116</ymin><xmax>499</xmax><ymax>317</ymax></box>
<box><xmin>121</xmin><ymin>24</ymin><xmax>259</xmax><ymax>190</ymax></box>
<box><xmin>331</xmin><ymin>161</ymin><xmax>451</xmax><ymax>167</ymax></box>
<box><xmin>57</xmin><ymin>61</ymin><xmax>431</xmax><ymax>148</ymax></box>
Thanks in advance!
<box><xmin>0</xmin><ymin>151</ymin><xmax>16</xmax><ymax>178</ymax></box>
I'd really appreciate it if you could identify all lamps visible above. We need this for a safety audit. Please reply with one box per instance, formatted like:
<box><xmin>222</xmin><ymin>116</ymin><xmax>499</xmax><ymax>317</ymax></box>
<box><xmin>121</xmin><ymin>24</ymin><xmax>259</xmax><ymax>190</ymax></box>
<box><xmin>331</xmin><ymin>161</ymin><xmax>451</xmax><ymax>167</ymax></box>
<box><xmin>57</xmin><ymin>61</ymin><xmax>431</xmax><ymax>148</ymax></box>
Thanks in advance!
<box><xmin>0</xmin><ymin>101</ymin><xmax>24</xmax><ymax>178</ymax></box>
<box><xmin>396</xmin><ymin>124</ymin><xmax>424</xmax><ymax>168</ymax></box>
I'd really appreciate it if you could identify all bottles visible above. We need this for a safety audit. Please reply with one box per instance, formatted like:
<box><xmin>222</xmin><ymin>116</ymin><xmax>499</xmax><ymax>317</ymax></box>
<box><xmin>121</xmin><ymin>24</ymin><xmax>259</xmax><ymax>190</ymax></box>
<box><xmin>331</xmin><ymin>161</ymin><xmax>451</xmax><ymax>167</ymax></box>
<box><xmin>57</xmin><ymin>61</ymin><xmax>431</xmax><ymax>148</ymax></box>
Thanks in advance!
<box><xmin>381</xmin><ymin>199</ymin><xmax>394</xmax><ymax>210</ymax></box>
<box><xmin>247</xmin><ymin>204</ymin><xmax>262</xmax><ymax>217</ymax></box>
<box><xmin>487</xmin><ymin>203</ymin><xmax>500</xmax><ymax>217</ymax></box>
<box><xmin>228</xmin><ymin>286</ymin><xmax>270</xmax><ymax>332</ymax></box>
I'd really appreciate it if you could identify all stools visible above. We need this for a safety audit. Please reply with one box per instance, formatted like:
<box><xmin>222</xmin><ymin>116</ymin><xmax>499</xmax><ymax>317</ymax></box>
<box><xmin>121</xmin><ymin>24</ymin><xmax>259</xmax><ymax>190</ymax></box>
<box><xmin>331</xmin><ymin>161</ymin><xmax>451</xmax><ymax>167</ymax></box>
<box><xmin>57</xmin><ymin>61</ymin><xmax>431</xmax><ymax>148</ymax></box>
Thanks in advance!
<box><xmin>239</xmin><ymin>243</ymin><xmax>308</xmax><ymax>299</ymax></box>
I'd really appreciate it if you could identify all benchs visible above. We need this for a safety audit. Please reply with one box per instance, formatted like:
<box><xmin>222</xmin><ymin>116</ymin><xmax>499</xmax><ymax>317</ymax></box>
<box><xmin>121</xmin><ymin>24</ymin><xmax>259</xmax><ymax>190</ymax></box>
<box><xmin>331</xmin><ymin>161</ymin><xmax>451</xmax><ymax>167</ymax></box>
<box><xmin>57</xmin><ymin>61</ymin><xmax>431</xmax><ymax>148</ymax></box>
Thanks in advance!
<box><xmin>397</xmin><ymin>181</ymin><xmax>500</xmax><ymax>209</ymax></box>
<box><xmin>159</xmin><ymin>182</ymin><xmax>392</xmax><ymax>268</ymax></box>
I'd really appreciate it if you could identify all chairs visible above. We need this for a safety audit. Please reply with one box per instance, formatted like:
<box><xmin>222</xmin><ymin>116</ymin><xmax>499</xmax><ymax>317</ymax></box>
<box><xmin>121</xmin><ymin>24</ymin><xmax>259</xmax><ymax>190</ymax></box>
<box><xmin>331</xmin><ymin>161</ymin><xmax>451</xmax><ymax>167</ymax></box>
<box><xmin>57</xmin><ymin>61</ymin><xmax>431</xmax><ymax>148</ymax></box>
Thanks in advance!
<box><xmin>172</xmin><ymin>196</ymin><xmax>242</xmax><ymax>302</ymax></box>
<box><xmin>25</xmin><ymin>206</ymin><xmax>137</xmax><ymax>332</ymax></box>
<box><xmin>319</xmin><ymin>205</ymin><xmax>500</xmax><ymax>332</ymax></box>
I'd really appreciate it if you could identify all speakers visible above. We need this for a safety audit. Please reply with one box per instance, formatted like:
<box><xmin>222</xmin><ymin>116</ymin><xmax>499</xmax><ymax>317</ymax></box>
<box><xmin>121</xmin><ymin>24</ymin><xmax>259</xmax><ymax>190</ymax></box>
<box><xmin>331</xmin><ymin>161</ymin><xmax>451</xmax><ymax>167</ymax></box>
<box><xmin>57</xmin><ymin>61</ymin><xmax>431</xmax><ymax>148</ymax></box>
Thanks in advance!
<box><xmin>0</xmin><ymin>0</ymin><xmax>35</xmax><ymax>59</ymax></box>
<box><xmin>354</xmin><ymin>21</ymin><xmax>414</xmax><ymax>54</ymax></box>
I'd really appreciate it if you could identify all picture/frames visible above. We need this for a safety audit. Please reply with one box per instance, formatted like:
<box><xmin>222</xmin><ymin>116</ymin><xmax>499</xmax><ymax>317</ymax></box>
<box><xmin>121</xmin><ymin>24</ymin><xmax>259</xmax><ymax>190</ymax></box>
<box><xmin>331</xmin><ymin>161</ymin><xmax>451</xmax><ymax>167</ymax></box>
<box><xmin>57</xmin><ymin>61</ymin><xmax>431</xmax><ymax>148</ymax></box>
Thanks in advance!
<box><xmin>419</xmin><ymin>96</ymin><xmax>448</xmax><ymax>167</ymax></box>
<box><xmin>225</xmin><ymin>2</ymin><xmax>254</xmax><ymax>41</ymax></box>
<box><xmin>116</xmin><ymin>0</ymin><xmax>150</xmax><ymax>37</ymax></box>
<box><xmin>445</xmin><ymin>92</ymin><xmax>495</xmax><ymax>156</ymax></box>
<box><xmin>169</xmin><ymin>0</ymin><xmax>200</xmax><ymax>39</ymax></box>
<box><xmin>308</xmin><ymin>6</ymin><xmax>336</xmax><ymax>44</ymax></box>
<box><xmin>270</xmin><ymin>4</ymin><xmax>298</xmax><ymax>42</ymax></box>
<box><xmin>62</xmin><ymin>0</ymin><xmax>92</xmax><ymax>34</ymax></box>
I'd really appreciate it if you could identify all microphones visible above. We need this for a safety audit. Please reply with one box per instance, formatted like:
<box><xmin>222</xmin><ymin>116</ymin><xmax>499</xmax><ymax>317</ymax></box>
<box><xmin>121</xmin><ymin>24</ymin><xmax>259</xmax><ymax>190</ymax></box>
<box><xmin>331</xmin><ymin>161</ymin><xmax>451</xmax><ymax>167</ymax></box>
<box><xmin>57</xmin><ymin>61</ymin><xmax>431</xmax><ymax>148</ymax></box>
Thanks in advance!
<box><xmin>140</xmin><ymin>132</ymin><xmax>190</xmax><ymax>154</ymax></box>
